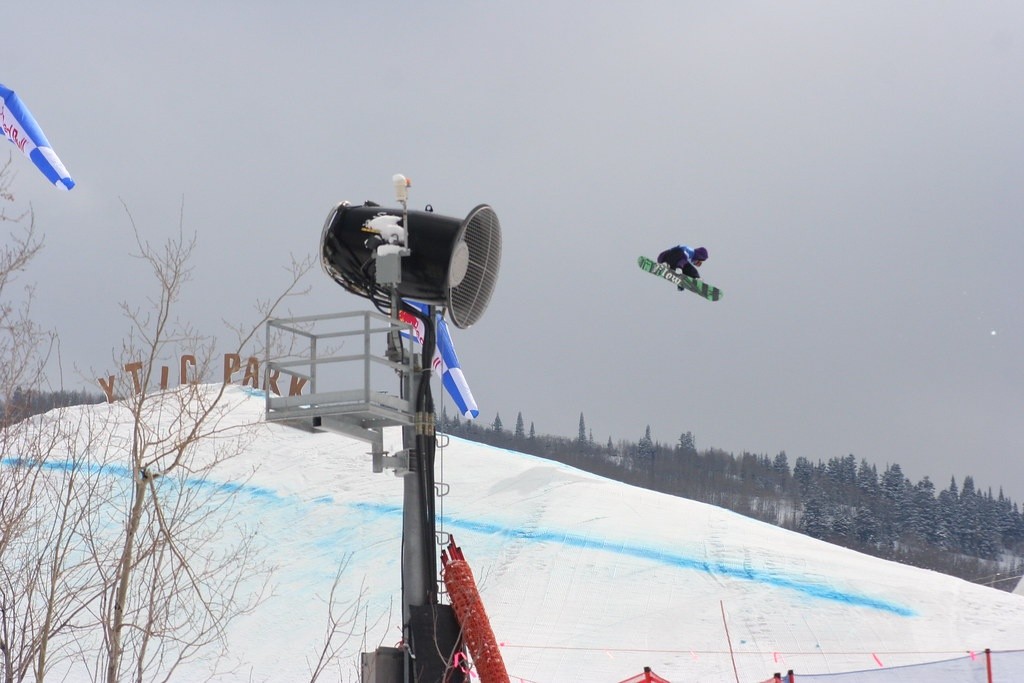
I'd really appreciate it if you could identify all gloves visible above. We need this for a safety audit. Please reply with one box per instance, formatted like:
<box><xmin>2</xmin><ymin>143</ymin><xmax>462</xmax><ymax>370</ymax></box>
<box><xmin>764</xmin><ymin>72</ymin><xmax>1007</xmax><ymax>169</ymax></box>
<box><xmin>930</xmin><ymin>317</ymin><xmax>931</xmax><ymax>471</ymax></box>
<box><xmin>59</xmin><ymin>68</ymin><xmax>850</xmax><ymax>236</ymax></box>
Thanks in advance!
<box><xmin>675</xmin><ymin>268</ymin><xmax>683</xmax><ymax>274</ymax></box>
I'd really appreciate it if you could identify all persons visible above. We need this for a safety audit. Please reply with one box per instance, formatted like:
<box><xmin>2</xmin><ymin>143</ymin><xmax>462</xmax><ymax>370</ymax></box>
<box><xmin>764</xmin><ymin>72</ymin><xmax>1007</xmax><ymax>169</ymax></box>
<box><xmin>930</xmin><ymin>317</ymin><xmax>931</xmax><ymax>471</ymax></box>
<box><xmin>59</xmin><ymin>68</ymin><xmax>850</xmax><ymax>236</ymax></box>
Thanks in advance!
<box><xmin>657</xmin><ymin>245</ymin><xmax>708</xmax><ymax>291</ymax></box>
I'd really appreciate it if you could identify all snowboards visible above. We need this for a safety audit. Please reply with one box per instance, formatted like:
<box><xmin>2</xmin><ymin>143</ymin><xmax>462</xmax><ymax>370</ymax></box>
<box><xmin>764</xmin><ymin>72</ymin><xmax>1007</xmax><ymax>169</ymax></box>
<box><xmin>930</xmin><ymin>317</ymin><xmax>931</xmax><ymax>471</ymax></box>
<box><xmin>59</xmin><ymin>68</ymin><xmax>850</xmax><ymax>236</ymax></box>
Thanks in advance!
<box><xmin>638</xmin><ymin>256</ymin><xmax>725</xmax><ymax>302</ymax></box>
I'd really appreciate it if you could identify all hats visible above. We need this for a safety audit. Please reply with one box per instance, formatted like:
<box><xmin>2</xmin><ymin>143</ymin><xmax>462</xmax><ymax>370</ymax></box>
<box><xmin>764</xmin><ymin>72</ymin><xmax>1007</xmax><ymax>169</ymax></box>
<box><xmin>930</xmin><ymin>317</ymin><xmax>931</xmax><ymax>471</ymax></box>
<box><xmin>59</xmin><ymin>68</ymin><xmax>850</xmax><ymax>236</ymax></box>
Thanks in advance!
<box><xmin>695</xmin><ymin>247</ymin><xmax>708</xmax><ymax>261</ymax></box>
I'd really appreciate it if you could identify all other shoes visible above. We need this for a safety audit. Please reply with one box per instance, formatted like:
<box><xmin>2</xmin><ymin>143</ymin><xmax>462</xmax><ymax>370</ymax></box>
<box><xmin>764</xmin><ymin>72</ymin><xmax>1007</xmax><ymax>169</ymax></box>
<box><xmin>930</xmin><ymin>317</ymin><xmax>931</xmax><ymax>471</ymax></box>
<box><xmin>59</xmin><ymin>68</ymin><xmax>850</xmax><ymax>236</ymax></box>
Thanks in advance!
<box><xmin>662</xmin><ymin>261</ymin><xmax>670</xmax><ymax>268</ymax></box>
